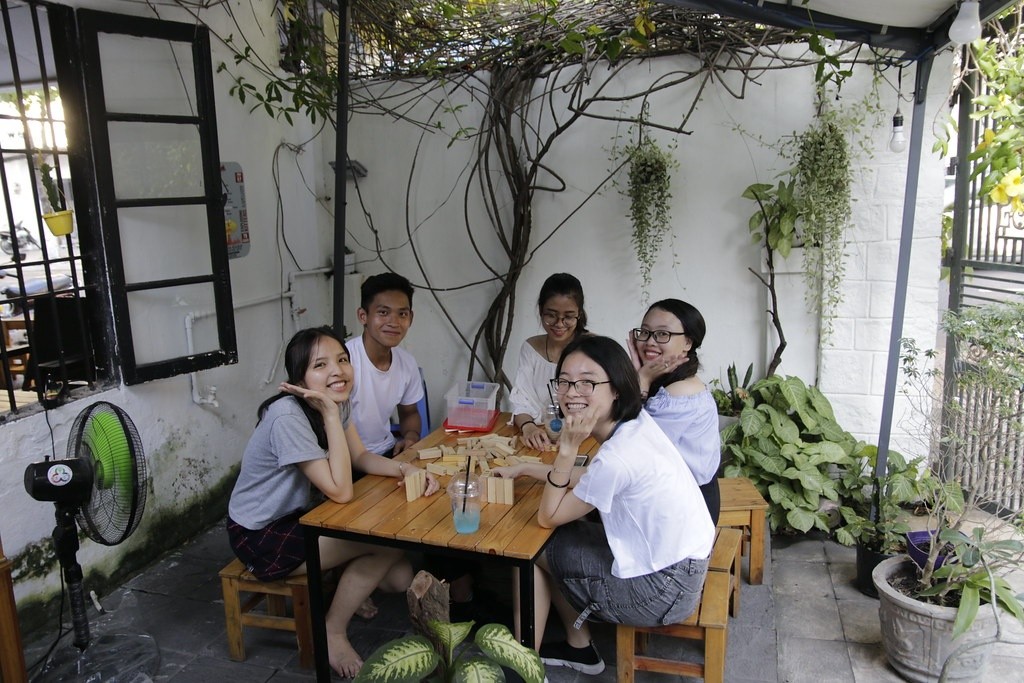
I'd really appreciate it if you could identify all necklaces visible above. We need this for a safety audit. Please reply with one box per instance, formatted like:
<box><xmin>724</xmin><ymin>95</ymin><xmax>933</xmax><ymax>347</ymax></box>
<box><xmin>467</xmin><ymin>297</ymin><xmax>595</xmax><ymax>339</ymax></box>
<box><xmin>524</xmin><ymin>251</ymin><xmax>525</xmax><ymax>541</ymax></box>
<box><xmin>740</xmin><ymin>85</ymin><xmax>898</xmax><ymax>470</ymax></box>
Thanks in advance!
<box><xmin>546</xmin><ymin>335</ymin><xmax>552</xmax><ymax>362</ymax></box>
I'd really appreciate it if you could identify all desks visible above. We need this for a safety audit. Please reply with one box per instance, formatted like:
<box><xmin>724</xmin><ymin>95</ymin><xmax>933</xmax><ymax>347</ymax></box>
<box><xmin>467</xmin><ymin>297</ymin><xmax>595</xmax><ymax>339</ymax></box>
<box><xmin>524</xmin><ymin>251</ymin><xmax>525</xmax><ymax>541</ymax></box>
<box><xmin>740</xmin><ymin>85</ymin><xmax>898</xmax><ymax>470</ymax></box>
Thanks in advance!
<box><xmin>0</xmin><ymin>309</ymin><xmax>34</xmax><ymax>366</ymax></box>
<box><xmin>299</xmin><ymin>412</ymin><xmax>601</xmax><ymax>683</ymax></box>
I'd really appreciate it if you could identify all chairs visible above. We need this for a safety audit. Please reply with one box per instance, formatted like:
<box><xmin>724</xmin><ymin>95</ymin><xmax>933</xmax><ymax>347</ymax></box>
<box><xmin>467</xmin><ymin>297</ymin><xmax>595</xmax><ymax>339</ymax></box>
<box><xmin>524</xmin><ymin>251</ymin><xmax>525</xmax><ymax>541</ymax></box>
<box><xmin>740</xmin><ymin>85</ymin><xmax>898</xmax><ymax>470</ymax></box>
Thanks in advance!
<box><xmin>389</xmin><ymin>366</ymin><xmax>431</xmax><ymax>439</ymax></box>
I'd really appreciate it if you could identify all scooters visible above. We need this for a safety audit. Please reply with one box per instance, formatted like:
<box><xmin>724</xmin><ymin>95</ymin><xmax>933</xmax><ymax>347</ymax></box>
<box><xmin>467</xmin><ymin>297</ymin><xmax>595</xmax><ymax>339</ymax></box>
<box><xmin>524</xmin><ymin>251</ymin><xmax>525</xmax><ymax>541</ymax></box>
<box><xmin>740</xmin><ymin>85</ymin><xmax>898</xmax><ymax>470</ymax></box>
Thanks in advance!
<box><xmin>0</xmin><ymin>270</ymin><xmax>74</xmax><ymax>343</ymax></box>
<box><xmin>0</xmin><ymin>221</ymin><xmax>42</xmax><ymax>256</ymax></box>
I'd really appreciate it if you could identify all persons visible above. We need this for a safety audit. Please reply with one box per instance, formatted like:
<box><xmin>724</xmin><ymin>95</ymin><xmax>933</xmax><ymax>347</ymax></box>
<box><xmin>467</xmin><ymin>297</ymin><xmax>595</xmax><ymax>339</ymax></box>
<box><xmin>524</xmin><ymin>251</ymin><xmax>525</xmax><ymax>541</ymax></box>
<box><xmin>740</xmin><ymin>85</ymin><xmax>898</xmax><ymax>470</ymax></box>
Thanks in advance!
<box><xmin>490</xmin><ymin>335</ymin><xmax>716</xmax><ymax>674</ymax></box>
<box><xmin>626</xmin><ymin>298</ymin><xmax>720</xmax><ymax>524</ymax></box>
<box><xmin>226</xmin><ymin>329</ymin><xmax>440</xmax><ymax>679</ymax></box>
<box><xmin>507</xmin><ymin>272</ymin><xmax>588</xmax><ymax>452</ymax></box>
<box><xmin>345</xmin><ymin>274</ymin><xmax>423</xmax><ymax>459</ymax></box>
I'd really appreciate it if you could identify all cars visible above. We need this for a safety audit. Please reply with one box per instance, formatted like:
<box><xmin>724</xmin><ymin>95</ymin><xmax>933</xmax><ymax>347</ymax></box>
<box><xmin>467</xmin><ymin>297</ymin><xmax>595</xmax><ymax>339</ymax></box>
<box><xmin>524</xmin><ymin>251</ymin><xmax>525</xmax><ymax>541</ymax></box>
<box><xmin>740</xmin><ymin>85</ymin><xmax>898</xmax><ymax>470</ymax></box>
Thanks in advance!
<box><xmin>943</xmin><ymin>174</ymin><xmax>1023</xmax><ymax>263</ymax></box>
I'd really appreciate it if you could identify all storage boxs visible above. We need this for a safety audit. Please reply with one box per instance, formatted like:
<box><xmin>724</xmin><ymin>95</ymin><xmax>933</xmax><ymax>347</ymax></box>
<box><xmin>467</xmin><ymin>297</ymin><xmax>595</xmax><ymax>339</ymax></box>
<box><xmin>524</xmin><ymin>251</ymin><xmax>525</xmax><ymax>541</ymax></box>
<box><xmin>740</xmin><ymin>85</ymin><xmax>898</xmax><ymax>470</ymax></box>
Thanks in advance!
<box><xmin>443</xmin><ymin>380</ymin><xmax>500</xmax><ymax>431</ymax></box>
<box><xmin>24</xmin><ymin>401</ymin><xmax>159</xmax><ymax>683</ymax></box>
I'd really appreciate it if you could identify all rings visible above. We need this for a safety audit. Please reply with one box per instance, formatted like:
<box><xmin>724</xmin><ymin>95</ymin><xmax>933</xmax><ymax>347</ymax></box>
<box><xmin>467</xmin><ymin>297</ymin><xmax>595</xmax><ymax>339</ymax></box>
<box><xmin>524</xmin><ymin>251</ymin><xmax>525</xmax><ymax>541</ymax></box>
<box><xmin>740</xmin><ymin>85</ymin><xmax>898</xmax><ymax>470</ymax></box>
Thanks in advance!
<box><xmin>665</xmin><ymin>364</ymin><xmax>668</xmax><ymax>368</ymax></box>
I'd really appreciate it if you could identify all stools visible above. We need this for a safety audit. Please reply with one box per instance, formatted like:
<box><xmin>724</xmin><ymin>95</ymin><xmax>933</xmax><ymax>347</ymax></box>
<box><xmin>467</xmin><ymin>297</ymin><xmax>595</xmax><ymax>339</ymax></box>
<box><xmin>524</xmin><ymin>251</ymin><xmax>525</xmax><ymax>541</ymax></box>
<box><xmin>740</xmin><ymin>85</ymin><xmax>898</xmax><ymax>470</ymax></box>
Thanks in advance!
<box><xmin>708</xmin><ymin>528</ymin><xmax>743</xmax><ymax>618</ymax></box>
<box><xmin>716</xmin><ymin>478</ymin><xmax>769</xmax><ymax>583</ymax></box>
<box><xmin>217</xmin><ymin>557</ymin><xmax>333</xmax><ymax>670</ymax></box>
<box><xmin>616</xmin><ymin>571</ymin><xmax>730</xmax><ymax>683</ymax></box>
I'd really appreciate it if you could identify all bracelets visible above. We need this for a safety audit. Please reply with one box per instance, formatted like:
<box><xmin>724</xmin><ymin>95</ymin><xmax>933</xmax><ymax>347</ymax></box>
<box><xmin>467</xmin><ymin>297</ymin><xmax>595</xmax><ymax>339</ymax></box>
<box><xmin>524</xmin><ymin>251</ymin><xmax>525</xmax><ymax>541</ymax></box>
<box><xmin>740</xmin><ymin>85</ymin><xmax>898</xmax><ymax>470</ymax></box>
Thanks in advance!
<box><xmin>400</xmin><ymin>460</ymin><xmax>410</xmax><ymax>478</ymax></box>
<box><xmin>547</xmin><ymin>471</ymin><xmax>570</xmax><ymax>487</ymax></box>
<box><xmin>521</xmin><ymin>420</ymin><xmax>536</xmax><ymax>432</ymax></box>
<box><xmin>552</xmin><ymin>466</ymin><xmax>573</xmax><ymax>473</ymax></box>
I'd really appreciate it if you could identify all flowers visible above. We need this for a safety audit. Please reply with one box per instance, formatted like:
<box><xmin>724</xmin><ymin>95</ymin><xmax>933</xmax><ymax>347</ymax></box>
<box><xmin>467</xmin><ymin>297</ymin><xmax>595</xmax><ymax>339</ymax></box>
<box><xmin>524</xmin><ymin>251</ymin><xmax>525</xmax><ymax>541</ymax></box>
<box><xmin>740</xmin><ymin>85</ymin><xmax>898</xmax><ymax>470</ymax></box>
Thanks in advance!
<box><xmin>897</xmin><ymin>290</ymin><xmax>1024</xmax><ymax>644</ymax></box>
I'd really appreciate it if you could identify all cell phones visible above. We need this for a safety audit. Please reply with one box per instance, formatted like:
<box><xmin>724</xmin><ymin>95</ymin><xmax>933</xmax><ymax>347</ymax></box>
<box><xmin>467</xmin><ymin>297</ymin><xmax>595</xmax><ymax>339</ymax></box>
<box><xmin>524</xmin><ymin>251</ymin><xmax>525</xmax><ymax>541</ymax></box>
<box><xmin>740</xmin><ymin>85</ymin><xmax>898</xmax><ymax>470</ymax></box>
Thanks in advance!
<box><xmin>574</xmin><ymin>456</ymin><xmax>588</xmax><ymax>467</ymax></box>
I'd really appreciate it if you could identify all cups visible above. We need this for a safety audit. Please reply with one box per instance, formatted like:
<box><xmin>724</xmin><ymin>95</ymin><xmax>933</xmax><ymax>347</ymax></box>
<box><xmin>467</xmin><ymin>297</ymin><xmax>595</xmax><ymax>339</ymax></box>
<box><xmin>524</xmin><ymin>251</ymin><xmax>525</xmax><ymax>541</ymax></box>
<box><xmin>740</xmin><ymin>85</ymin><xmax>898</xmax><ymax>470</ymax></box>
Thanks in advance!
<box><xmin>447</xmin><ymin>471</ymin><xmax>483</xmax><ymax>533</ymax></box>
<box><xmin>542</xmin><ymin>394</ymin><xmax>563</xmax><ymax>443</ymax></box>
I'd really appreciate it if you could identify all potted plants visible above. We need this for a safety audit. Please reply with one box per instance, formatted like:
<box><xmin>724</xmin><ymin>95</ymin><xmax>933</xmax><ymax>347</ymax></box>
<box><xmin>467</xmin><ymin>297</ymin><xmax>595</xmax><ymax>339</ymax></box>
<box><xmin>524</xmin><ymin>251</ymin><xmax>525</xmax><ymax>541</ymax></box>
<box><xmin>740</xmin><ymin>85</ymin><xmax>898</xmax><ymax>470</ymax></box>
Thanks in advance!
<box><xmin>594</xmin><ymin>40</ymin><xmax>963</xmax><ymax>598</ymax></box>
<box><xmin>39</xmin><ymin>163</ymin><xmax>74</xmax><ymax>236</ymax></box>
<box><xmin>329</xmin><ymin>247</ymin><xmax>355</xmax><ymax>274</ymax></box>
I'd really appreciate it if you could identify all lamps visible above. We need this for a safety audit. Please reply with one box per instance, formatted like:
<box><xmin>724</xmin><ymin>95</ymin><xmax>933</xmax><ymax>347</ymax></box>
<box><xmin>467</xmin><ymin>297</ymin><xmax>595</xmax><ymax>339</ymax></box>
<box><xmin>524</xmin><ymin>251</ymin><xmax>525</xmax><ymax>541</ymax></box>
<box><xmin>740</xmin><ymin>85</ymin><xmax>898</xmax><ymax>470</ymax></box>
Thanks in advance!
<box><xmin>329</xmin><ymin>160</ymin><xmax>368</xmax><ymax>181</ymax></box>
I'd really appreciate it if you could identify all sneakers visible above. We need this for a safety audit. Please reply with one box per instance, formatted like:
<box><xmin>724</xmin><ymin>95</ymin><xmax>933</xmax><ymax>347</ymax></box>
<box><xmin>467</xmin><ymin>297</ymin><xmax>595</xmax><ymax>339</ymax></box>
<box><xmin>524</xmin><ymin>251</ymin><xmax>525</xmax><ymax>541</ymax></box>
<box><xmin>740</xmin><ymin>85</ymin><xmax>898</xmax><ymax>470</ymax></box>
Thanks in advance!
<box><xmin>539</xmin><ymin>638</ymin><xmax>606</xmax><ymax>675</ymax></box>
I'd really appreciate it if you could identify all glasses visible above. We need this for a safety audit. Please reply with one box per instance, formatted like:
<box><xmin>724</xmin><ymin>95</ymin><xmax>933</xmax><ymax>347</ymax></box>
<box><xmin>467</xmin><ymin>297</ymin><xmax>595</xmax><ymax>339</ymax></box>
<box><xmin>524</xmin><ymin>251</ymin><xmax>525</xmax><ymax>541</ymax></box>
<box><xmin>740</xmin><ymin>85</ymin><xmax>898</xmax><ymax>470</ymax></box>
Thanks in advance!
<box><xmin>634</xmin><ymin>327</ymin><xmax>685</xmax><ymax>345</ymax></box>
<box><xmin>550</xmin><ymin>378</ymin><xmax>609</xmax><ymax>397</ymax></box>
<box><xmin>542</xmin><ymin>312</ymin><xmax>580</xmax><ymax>328</ymax></box>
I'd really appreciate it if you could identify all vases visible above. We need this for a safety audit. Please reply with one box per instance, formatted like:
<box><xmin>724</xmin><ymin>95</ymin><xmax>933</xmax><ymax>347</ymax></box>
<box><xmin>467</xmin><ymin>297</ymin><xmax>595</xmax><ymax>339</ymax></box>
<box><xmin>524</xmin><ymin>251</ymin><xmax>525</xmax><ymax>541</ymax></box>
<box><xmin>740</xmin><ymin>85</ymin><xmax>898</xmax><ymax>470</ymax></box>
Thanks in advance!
<box><xmin>872</xmin><ymin>554</ymin><xmax>1007</xmax><ymax>683</ymax></box>
